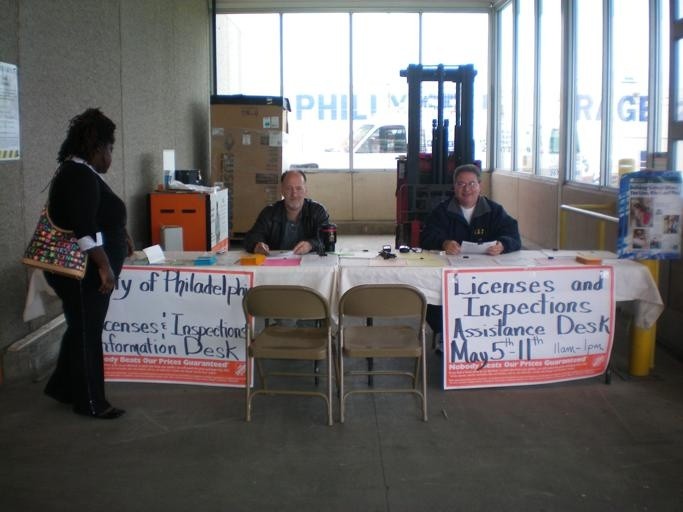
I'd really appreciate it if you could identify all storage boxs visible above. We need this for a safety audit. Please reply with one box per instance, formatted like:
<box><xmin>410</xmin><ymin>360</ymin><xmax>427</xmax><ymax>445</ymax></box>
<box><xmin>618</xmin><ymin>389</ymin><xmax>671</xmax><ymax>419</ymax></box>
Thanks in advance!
<box><xmin>210</xmin><ymin>95</ymin><xmax>289</xmax><ymax>239</ymax></box>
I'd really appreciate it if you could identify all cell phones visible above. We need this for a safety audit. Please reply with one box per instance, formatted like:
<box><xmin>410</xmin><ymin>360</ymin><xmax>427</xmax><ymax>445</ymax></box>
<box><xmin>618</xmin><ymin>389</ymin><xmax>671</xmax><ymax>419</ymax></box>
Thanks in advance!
<box><xmin>383</xmin><ymin>245</ymin><xmax>391</xmax><ymax>251</ymax></box>
<box><xmin>400</xmin><ymin>246</ymin><xmax>410</xmax><ymax>252</ymax></box>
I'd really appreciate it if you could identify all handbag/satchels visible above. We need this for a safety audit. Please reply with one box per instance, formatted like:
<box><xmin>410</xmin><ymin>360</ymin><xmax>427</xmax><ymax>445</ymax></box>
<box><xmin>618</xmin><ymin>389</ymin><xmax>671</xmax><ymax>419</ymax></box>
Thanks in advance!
<box><xmin>20</xmin><ymin>165</ymin><xmax>88</xmax><ymax>282</ymax></box>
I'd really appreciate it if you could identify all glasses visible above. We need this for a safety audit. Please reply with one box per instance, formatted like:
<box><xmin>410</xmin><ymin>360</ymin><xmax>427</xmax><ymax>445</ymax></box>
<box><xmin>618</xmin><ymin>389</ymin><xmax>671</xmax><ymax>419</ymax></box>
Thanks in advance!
<box><xmin>454</xmin><ymin>182</ymin><xmax>478</xmax><ymax>188</ymax></box>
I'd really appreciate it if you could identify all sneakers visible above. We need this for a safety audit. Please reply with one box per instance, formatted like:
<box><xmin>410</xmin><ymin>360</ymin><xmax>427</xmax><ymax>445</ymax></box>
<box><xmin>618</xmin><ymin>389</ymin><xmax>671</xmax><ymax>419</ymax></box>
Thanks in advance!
<box><xmin>433</xmin><ymin>332</ymin><xmax>443</xmax><ymax>355</ymax></box>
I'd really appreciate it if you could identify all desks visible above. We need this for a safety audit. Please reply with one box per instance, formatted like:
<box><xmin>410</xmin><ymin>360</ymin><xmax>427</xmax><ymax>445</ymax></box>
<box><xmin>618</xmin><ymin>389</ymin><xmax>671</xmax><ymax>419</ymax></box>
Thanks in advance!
<box><xmin>122</xmin><ymin>249</ymin><xmax>659</xmax><ymax>377</ymax></box>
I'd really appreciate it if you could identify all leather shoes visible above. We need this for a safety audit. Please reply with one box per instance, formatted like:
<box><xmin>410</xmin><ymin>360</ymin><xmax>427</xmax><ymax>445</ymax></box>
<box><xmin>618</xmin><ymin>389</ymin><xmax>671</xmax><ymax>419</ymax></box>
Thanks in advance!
<box><xmin>43</xmin><ymin>385</ymin><xmax>126</xmax><ymax>419</ymax></box>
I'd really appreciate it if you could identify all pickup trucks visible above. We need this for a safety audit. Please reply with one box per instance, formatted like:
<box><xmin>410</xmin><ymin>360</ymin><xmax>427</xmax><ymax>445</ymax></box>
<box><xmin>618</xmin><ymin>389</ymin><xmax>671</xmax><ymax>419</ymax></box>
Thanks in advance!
<box><xmin>289</xmin><ymin>121</ymin><xmax>455</xmax><ymax>170</ymax></box>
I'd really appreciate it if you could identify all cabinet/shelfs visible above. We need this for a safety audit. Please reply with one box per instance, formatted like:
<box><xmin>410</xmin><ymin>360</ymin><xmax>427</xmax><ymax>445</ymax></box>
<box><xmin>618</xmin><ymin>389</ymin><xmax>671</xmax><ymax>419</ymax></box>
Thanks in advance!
<box><xmin>151</xmin><ymin>188</ymin><xmax>228</xmax><ymax>251</ymax></box>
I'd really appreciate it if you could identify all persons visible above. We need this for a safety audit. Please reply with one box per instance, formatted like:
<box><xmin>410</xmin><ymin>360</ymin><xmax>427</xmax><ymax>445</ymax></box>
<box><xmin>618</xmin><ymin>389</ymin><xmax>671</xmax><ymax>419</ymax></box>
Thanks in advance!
<box><xmin>631</xmin><ymin>228</ymin><xmax>645</xmax><ymax>249</ymax></box>
<box><xmin>632</xmin><ymin>202</ymin><xmax>652</xmax><ymax>226</ymax></box>
<box><xmin>41</xmin><ymin>106</ymin><xmax>135</xmax><ymax>419</ymax></box>
<box><xmin>418</xmin><ymin>164</ymin><xmax>522</xmax><ymax>354</ymax></box>
<box><xmin>243</xmin><ymin>169</ymin><xmax>336</xmax><ymax>258</ymax></box>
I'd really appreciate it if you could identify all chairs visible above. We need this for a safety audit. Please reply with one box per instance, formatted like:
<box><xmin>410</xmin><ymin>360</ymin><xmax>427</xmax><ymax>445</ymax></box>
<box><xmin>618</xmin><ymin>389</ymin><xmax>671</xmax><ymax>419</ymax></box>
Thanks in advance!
<box><xmin>242</xmin><ymin>284</ymin><xmax>427</xmax><ymax>425</ymax></box>
